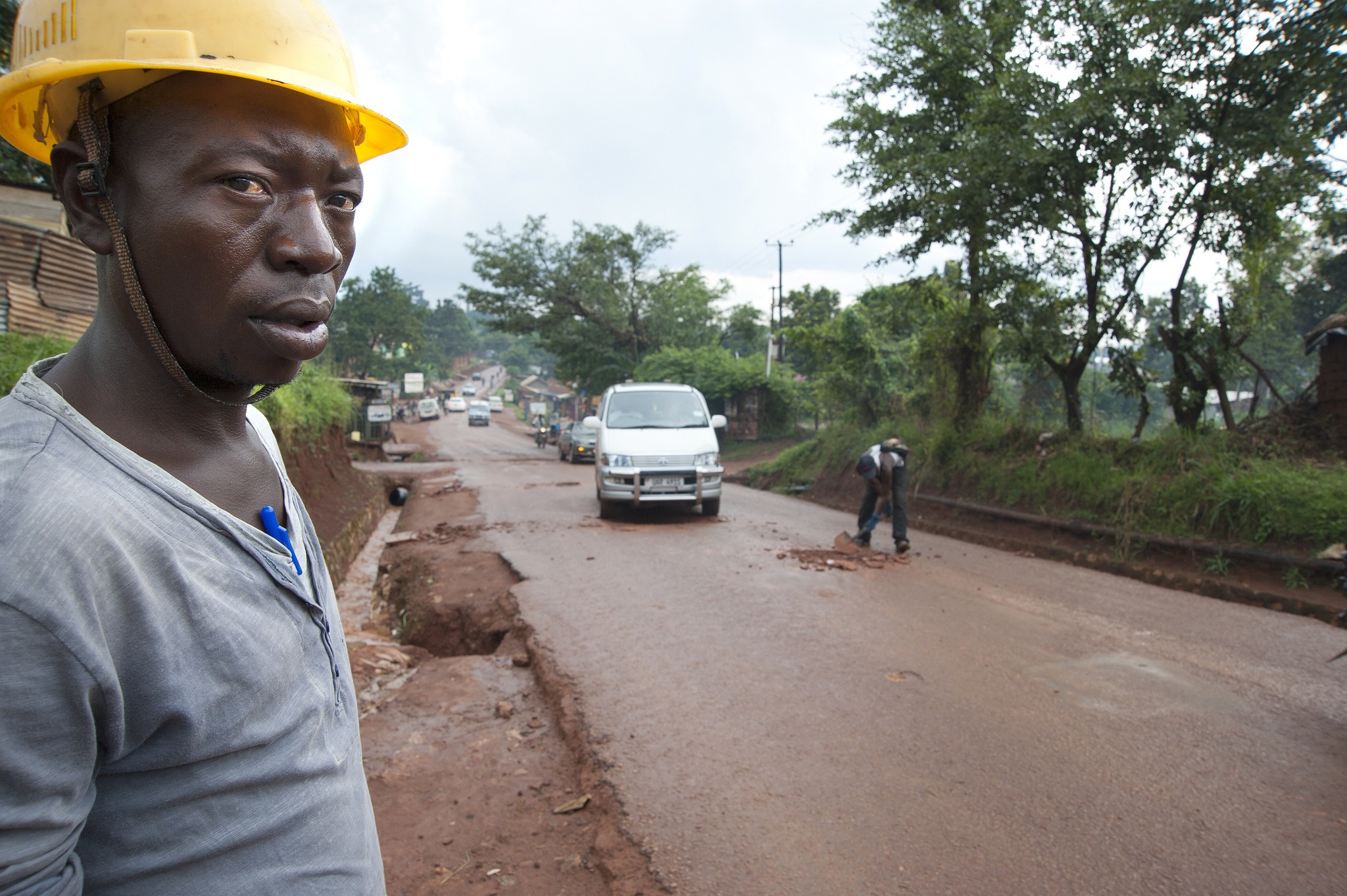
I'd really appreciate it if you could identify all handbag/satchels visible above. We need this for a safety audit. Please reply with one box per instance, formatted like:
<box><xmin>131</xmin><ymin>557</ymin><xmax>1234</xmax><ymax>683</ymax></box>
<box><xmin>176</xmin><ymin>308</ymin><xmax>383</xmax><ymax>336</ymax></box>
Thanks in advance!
<box><xmin>879</xmin><ymin>439</ymin><xmax>908</xmax><ymax>457</ymax></box>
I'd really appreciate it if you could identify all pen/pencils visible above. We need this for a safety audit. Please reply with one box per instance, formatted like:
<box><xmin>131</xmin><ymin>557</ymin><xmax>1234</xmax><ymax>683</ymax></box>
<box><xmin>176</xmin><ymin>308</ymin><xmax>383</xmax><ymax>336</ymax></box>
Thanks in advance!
<box><xmin>260</xmin><ymin>504</ymin><xmax>304</xmax><ymax>576</ymax></box>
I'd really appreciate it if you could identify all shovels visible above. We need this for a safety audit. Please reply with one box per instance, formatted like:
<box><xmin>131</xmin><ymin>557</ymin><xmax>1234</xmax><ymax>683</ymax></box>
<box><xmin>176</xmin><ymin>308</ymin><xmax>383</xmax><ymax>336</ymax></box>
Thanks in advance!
<box><xmin>834</xmin><ymin>514</ymin><xmax>888</xmax><ymax>555</ymax></box>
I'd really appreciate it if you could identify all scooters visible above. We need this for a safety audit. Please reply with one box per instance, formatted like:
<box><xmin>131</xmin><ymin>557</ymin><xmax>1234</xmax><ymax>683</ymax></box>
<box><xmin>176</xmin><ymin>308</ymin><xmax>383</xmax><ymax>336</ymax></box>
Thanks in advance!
<box><xmin>534</xmin><ymin>426</ymin><xmax>552</xmax><ymax>449</ymax></box>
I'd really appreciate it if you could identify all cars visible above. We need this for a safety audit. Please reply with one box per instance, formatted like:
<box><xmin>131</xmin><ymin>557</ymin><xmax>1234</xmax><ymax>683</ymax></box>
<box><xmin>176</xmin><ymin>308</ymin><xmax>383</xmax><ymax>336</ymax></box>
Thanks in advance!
<box><xmin>463</xmin><ymin>383</ymin><xmax>476</xmax><ymax>396</ymax></box>
<box><xmin>472</xmin><ymin>372</ymin><xmax>481</xmax><ymax>380</ymax></box>
<box><xmin>557</xmin><ymin>420</ymin><xmax>599</xmax><ymax>464</ymax></box>
<box><xmin>446</xmin><ymin>396</ymin><xmax>467</xmax><ymax>412</ymax></box>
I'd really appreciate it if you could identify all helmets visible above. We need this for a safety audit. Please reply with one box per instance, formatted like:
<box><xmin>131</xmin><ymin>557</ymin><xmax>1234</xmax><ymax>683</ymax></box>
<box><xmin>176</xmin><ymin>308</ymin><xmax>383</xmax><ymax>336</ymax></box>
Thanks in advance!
<box><xmin>0</xmin><ymin>0</ymin><xmax>408</xmax><ymax>163</ymax></box>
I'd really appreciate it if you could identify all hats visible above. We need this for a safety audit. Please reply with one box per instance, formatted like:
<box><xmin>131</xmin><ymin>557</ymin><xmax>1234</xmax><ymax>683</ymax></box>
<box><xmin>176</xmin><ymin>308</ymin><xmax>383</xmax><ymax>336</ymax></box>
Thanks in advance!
<box><xmin>857</xmin><ymin>454</ymin><xmax>876</xmax><ymax>478</ymax></box>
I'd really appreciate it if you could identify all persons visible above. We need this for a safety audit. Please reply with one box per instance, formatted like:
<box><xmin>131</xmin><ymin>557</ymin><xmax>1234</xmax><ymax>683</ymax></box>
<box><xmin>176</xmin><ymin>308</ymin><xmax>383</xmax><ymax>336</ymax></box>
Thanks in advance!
<box><xmin>394</xmin><ymin>366</ymin><xmax>561</xmax><ymax>442</ymax></box>
<box><xmin>853</xmin><ymin>438</ymin><xmax>909</xmax><ymax>553</ymax></box>
<box><xmin>0</xmin><ymin>0</ymin><xmax>410</xmax><ymax>896</ymax></box>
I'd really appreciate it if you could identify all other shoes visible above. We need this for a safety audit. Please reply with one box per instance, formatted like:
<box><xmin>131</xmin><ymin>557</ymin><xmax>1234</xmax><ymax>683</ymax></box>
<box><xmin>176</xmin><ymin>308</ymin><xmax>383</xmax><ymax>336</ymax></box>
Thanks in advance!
<box><xmin>895</xmin><ymin>539</ymin><xmax>909</xmax><ymax>554</ymax></box>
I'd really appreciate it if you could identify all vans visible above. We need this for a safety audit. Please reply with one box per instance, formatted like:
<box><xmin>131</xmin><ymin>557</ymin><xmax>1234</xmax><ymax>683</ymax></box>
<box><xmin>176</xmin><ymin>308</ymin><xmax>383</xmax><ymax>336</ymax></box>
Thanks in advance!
<box><xmin>487</xmin><ymin>396</ymin><xmax>504</xmax><ymax>412</ymax></box>
<box><xmin>467</xmin><ymin>400</ymin><xmax>492</xmax><ymax>426</ymax></box>
<box><xmin>418</xmin><ymin>398</ymin><xmax>440</xmax><ymax>422</ymax></box>
<box><xmin>583</xmin><ymin>380</ymin><xmax>729</xmax><ymax>516</ymax></box>
